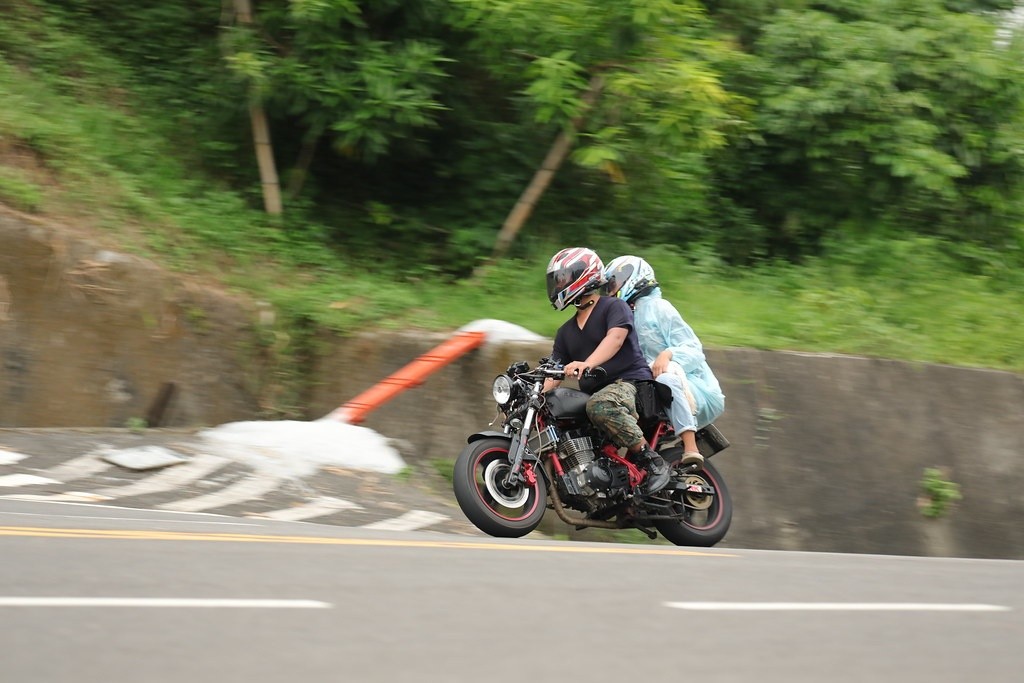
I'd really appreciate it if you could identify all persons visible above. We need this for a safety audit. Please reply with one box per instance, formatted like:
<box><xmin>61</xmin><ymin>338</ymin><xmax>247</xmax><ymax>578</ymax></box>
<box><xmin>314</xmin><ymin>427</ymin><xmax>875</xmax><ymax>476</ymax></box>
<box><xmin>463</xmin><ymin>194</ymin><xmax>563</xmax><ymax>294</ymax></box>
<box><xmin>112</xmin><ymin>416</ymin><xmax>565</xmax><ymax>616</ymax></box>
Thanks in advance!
<box><xmin>540</xmin><ymin>247</ymin><xmax>673</xmax><ymax>531</ymax></box>
<box><xmin>604</xmin><ymin>255</ymin><xmax>726</xmax><ymax>473</ymax></box>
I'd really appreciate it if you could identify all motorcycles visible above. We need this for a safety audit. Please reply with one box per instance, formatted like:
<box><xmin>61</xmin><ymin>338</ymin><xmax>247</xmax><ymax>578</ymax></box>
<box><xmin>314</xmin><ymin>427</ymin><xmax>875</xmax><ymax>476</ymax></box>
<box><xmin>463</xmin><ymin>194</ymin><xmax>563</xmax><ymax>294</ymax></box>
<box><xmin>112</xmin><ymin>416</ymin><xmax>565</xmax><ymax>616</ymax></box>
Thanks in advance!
<box><xmin>451</xmin><ymin>356</ymin><xmax>735</xmax><ymax>546</ymax></box>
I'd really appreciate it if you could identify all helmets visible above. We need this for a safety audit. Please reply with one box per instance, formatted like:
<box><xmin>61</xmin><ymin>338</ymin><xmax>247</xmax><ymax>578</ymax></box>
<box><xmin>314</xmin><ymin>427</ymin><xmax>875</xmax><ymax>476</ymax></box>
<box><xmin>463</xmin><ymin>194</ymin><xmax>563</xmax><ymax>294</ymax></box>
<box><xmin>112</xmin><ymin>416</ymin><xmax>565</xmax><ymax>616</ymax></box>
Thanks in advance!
<box><xmin>604</xmin><ymin>254</ymin><xmax>659</xmax><ymax>304</ymax></box>
<box><xmin>545</xmin><ymin>246</ymin><xmax>606</xmax><ymax>313</ymax></box>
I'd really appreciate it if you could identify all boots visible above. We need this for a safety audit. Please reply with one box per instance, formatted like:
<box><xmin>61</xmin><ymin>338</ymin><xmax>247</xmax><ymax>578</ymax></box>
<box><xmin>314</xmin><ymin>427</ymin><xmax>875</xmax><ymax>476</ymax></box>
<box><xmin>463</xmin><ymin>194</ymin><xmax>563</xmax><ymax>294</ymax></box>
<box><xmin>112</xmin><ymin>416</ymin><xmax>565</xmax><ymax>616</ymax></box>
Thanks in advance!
<box><xmin>639</xmin><ymin>447</ymin><xmax>673</xmax><ymax>496</ymax></box>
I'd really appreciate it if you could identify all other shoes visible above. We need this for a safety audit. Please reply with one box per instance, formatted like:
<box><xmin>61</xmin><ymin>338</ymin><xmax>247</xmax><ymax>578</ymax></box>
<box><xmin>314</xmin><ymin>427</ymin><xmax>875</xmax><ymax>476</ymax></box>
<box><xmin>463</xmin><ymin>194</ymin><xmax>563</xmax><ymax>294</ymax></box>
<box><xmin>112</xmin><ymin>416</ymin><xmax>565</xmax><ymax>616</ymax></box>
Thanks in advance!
<box><xmin>680</xmin><ymin>452</ymin><xmax>704</xmax><ymax>473</ymax></box>
